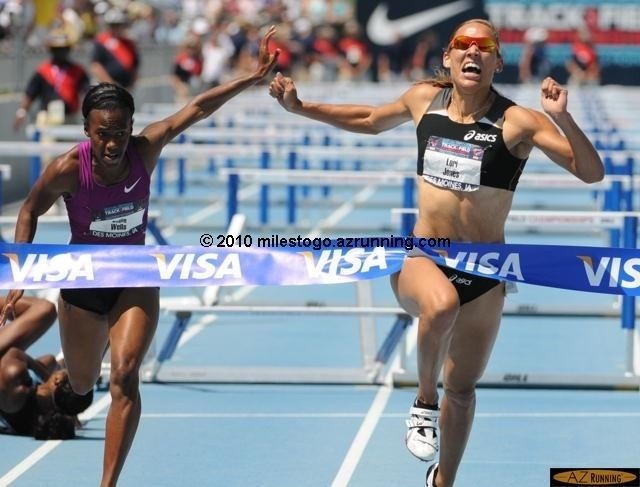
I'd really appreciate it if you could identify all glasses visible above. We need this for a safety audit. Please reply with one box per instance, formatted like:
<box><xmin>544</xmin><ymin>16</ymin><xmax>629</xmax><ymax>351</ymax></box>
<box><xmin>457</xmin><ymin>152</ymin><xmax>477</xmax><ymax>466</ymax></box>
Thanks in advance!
<box><xmin>447</xmin><ymin>34</ymin><xmax>497</xmax><ymax>53</ymax></box>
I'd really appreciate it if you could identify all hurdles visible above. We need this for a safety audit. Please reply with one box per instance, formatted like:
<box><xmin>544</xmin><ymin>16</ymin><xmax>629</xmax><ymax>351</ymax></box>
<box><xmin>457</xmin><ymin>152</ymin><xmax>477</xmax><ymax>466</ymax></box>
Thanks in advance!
<box><xmin>1</xmin><ymin>82</ymin><xmax>639</xmax><ymax>389</ymax></box>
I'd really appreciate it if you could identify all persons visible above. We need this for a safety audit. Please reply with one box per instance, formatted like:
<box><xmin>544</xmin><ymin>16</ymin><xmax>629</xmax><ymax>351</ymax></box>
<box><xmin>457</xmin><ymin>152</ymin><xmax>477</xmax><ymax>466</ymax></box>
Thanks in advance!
<box><xmin>0</xmin><ymin>24</ymin><xmax>281</xmax><ymax>487</ymax></box>
<box><xmin>269</xmin><ymin>19</ymin><xmax>605</xmax><ymax>487</ymax></box>
<box><xmin>0</xmin><ymin>0</ymin><xmax>639</xmax><ymax>135</ymax></box>
<box><xmin>0</xmin><ymin>297</ymin><xmax>93</xmax><ymax>439</ymax></box>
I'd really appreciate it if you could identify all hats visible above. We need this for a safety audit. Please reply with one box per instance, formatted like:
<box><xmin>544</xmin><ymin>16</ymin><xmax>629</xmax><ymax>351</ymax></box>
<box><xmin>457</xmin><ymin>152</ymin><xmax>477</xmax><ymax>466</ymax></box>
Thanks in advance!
<box><xmin>42</xmin><ymin>9</ymin><xmax>130</xmax><ymax>49</ymax></box>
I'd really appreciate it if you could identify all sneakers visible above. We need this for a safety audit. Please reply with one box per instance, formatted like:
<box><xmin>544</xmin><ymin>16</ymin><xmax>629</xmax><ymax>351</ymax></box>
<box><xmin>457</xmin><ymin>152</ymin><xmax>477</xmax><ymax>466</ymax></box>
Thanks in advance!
<box><xmin>424</xmin><ymin>461</ymin><xmax>440</xmax><ymax>487</ymax></box>
<box><xmin>406</xmin><ymin>393</ymin><xmax>441</xmax><ymax>461</ymax></box>
